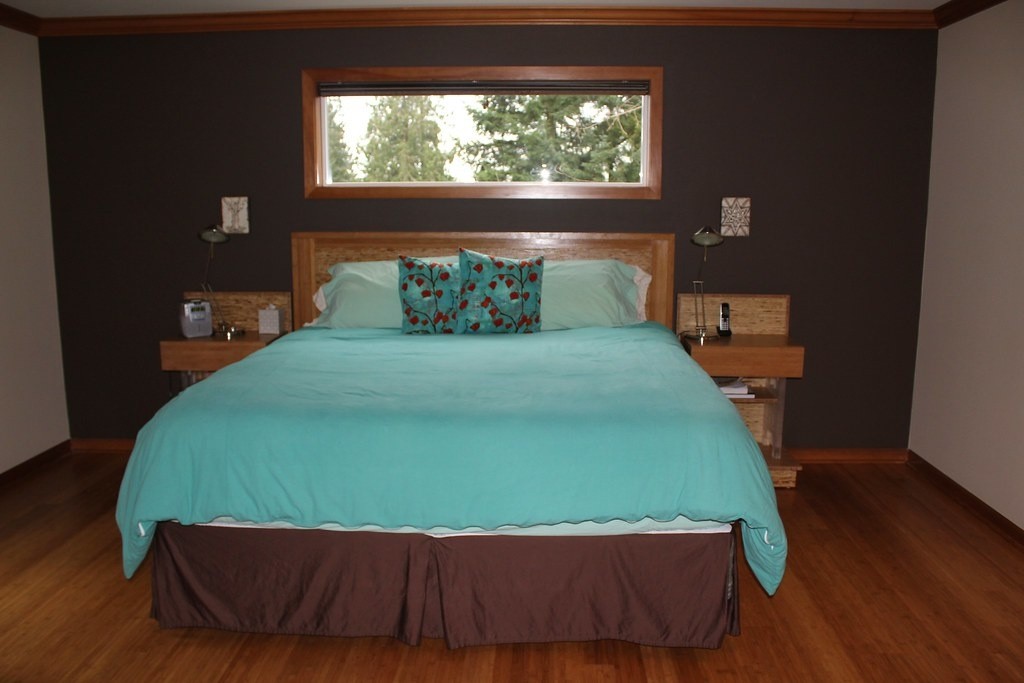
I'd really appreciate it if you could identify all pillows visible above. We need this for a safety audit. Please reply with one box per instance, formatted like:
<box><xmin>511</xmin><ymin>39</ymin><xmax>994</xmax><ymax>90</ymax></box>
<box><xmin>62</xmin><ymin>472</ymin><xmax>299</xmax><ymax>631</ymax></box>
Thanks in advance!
<box><xmin>543</xmin><ymin>258</ymin><xmax>653</xmax><ymax>331</ymax></box>
<box><xmin>455</xmin><ymin>247</ymin><xmax>544</xmax><ymax>334</ymax></box>
<box><xmin>398</xmin><ymin>254</ymin><xmax>460</xmax><ymax>336</ymax></box>
<box><xmin>303</xmin><ymin>256</ymin><xmax>461</xmax><ymax>330</ymax></box>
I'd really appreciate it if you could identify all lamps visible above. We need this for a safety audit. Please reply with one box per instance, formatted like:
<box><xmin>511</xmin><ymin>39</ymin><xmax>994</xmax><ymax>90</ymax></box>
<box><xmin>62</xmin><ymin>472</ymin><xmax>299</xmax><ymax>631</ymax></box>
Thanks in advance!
<box><xmin>682</xmin><ymin>225</ymin><xmax>723</xmax><ymax>342</ymax></box>
<box><xmin>196</xmin><ymin>228</ymin><xmax>246</xmax><ymax>341</ymax></box>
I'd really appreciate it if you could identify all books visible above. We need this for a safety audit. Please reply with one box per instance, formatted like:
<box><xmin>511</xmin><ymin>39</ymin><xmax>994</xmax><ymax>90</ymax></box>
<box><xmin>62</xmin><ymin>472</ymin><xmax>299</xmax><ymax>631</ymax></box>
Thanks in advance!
<box><xmin>713</xmin><ymin>377</ymin><xmax>756</xmax><ymax>399</ymax></box>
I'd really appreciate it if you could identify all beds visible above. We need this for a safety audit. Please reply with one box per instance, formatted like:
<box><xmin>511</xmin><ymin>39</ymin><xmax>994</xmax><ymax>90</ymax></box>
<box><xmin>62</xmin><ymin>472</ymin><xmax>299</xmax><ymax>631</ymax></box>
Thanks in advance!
<box><xmin>116</xmin><ymin>229</ymin><xmax>791</xmax><ymax>650</ymax></box>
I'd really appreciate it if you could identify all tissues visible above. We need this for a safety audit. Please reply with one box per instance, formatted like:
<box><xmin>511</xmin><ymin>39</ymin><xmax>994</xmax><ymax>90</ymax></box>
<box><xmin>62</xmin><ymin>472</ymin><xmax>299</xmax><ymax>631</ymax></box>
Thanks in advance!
<box><xmin>258</xmin><ymin>304</ymin><xmax>284</xmax><ymax>335</ymax></box>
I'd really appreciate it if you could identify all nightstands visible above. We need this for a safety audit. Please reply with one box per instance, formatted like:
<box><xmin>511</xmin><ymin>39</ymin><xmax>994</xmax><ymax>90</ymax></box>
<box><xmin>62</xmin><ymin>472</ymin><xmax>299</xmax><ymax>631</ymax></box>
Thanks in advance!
<box><xmin>159</xmin><ymin>290</ymin><xmax>293</xmax><ymax>389</ymax></box>
<box><xmin>675</xmin><ymin>293</ymin><xmax>804</xmax><ymax>488</ymax></box>
